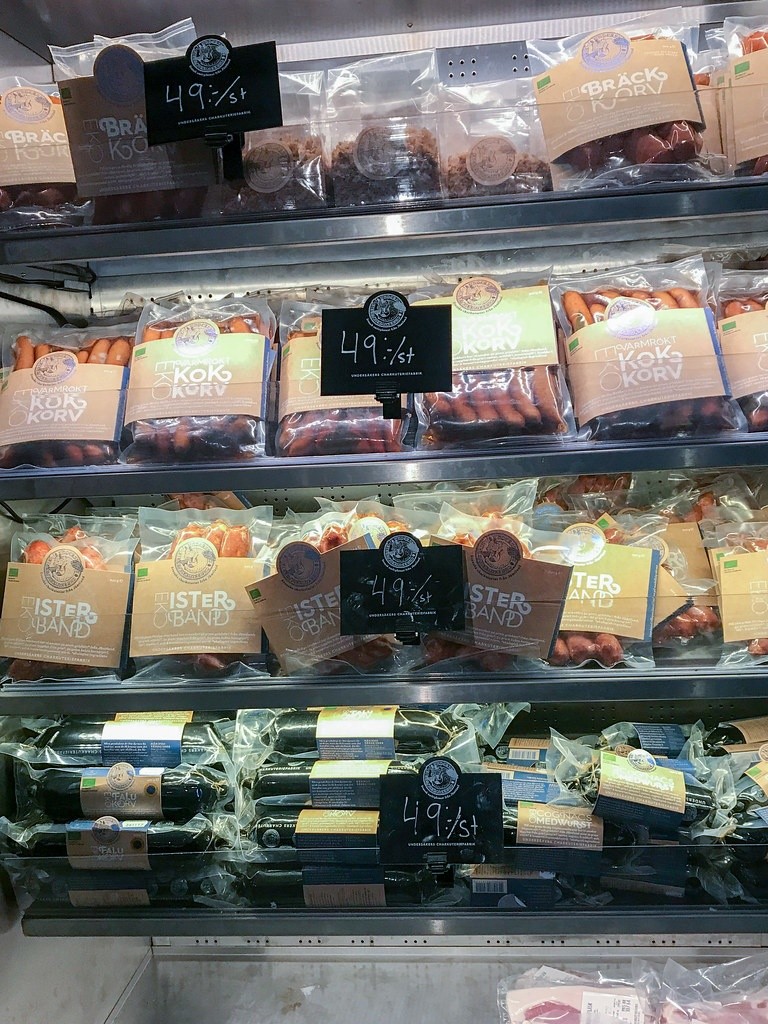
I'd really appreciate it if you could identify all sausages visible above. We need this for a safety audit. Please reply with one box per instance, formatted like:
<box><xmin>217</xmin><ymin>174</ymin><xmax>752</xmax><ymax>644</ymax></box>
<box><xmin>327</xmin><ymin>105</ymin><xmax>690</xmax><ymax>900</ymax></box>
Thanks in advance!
<box><xmin>0</xmin><ymin>23</ymin><xmax>768</xmax><ymax>671</ymax></box>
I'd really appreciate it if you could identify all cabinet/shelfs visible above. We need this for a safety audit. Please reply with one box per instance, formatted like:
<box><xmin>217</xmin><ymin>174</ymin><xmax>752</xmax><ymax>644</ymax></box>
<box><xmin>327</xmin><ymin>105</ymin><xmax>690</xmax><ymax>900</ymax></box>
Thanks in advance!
<box><xmin>1</xmin><ymin>83</ymin><xmax>768</xmax><ymax>938</ymax></box>
